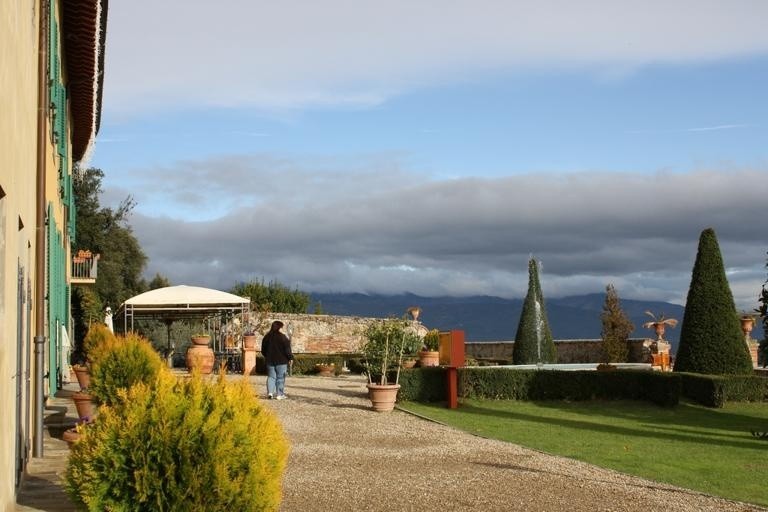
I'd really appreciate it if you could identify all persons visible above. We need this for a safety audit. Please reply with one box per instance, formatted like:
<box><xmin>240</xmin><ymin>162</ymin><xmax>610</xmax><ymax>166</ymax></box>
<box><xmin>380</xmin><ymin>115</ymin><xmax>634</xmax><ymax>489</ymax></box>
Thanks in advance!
<box><xmin>261</xmin><ymin>320</ymin><xmax>294</xmax><ymax>400</ymax></box>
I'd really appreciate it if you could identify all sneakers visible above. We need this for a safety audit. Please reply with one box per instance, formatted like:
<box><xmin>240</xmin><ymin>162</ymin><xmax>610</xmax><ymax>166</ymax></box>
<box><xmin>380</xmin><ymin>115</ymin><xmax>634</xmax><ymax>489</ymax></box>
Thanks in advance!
<box><xmin>268</xmin><ymin>392</ymin><xmax>273</xmax><ymax>399</ymax></box>
<box><xmin>277</xmin><ymin>394</ymin><xmax>288</xmax><ymax>400</ymax></box>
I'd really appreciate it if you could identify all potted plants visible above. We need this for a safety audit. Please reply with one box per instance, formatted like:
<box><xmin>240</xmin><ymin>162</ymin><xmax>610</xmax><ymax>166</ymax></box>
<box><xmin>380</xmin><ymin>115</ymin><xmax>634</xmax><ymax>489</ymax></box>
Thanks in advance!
<box><xmin>360</xmin><ymin>316</ymin><xmax>414</xmax><ymax>413</ymax></box>
<box><xmin>417</xmin><ymin>328</ymin><xmax>440</xmax><ymax>369</ymax></box>
<box><xmin>316</xmin><ymin>360</ymin><xmax>334</xmax><ymax>375</ymax></box>
<box><xmin>191</xmin><ymin>332</ymin><xmax>211</xmax><ymax>345</ymax></box>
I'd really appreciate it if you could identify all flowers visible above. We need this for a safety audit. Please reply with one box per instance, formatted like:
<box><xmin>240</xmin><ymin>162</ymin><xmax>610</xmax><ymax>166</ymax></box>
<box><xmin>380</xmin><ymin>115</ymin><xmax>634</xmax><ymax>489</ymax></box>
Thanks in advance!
<box><xmin>71</xmin><ymin>416</ymin><xmax>89</xmax><ymax>431</ymax></box>
<box><xmin>78</xmin><ymin>357</ymin><xmax>91</xmax><ymax>366</ymax></box>
<box><xmin>243</xmin><ymin>331</ymin><xmax>257</xmax><ymax>336</ymax></box>
<box><xmin>80</xmin><ymin>385</ymin><xmax>91</xmax><ymax>391</ymax></box>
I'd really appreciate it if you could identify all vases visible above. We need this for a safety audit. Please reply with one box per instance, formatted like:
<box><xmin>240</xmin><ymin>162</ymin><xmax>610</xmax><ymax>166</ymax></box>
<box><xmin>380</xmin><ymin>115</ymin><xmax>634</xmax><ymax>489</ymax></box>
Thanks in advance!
<box><xmin>72</xmin><ymin>392</ymin><xmax>98</xmax><ymax>424</ymax></box>
<box><xmin>245</xmin><ymin>336</ymin><xmax>257</xmax><ymax>347</ymax></box>
<box><xmin>63</xmin><ymin>428</ymin><xmax>82</xmax><ymax>449</ymax></box>
<box><xmin>186</xmin><ymin>345</ymin><xmax>216</xmax><ymax>373</ymax></box>
<box><xmin>73</xmin><ymin>364</ymin><xmax>91</xmax><ymax>388</ymax></box>
